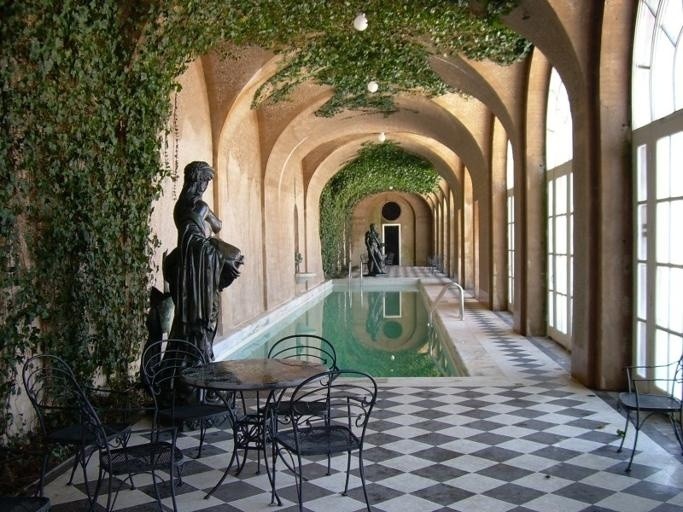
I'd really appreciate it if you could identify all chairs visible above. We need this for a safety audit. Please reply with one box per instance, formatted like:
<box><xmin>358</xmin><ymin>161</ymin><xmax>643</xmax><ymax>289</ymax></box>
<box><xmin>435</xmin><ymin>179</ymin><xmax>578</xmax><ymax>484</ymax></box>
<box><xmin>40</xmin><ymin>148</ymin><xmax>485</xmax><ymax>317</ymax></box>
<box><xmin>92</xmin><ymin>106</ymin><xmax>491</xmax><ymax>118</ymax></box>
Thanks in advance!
<box><xmin>270</xmin><ymin>370</ymin><xmax>377</xmax><ymax>512</ymax></box>
<box><xmin>22</xmin><ymin>354</ymin><xmax>136</xmax><ymax>505</ymax></box>
<box><xmin>255</xmin><ymin>334</ymin><xmax>340</xmax><ymax>475</ymax></box>
<box><xmin>617</xmin><ymin>354</ymin><xmax>683</xmax><ymax>472</ymax></box>
<box><xmin>360</xmin><ymin>253</ymin><xmax>442</xmax><ymax>274</ymax></box>
<box><xmin>142</xmin><ymin>339</ymin><xmax>241</xmax><ymax>485</ymax></box>
<box><xmin>72</xmin><ymin>391</ymin><xmax>183</xmax><ymax>512</ymax></box>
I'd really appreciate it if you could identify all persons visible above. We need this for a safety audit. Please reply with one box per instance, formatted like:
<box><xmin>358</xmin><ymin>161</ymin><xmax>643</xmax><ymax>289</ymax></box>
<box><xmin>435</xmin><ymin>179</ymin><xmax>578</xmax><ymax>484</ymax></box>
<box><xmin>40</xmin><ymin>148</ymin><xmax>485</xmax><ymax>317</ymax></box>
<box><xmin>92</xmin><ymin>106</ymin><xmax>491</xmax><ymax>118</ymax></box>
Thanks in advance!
<box><xmin>365</xmin><ymin>291</ymin><xmax>387</xmax><ymax>342</ymax></box>
<box><xmin>160</xmin><ymin>160</ymin><xmax>226</xmax><ymax>403</ymax></box>
<box><xmin>364</xmin><ymin>223</ymin><xmax>386</xmax><ymax>275</ymax></box>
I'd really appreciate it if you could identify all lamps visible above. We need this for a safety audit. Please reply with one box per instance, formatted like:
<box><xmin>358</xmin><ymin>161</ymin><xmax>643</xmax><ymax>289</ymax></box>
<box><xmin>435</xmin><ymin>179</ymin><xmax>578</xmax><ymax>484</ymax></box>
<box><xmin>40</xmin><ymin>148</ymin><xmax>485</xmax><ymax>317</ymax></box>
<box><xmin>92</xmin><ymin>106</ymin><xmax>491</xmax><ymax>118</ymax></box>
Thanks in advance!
<box><xmin>354</xmin><ymin>12</ymin><xmax>369</xmax><ymax>32</ymax></box>
<box><xmin>368</xmin><ymin>81</ymin><xmax>378</xmax><ymax>92</ymax></box>
<box><xmin>378</xmin><ymin>131</ymin><xmax>386</xmax><ymax>141</ymax></box>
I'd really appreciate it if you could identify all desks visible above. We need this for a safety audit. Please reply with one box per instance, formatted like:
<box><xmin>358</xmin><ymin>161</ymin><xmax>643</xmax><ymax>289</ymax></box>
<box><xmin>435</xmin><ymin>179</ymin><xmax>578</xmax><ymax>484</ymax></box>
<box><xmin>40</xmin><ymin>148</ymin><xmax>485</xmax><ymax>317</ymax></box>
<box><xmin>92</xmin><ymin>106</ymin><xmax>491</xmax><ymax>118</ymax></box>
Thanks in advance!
<box><xmin>180</xmin><ymin>359</ymin><xmax>330</xmax><ymax>506</ymax></box>
